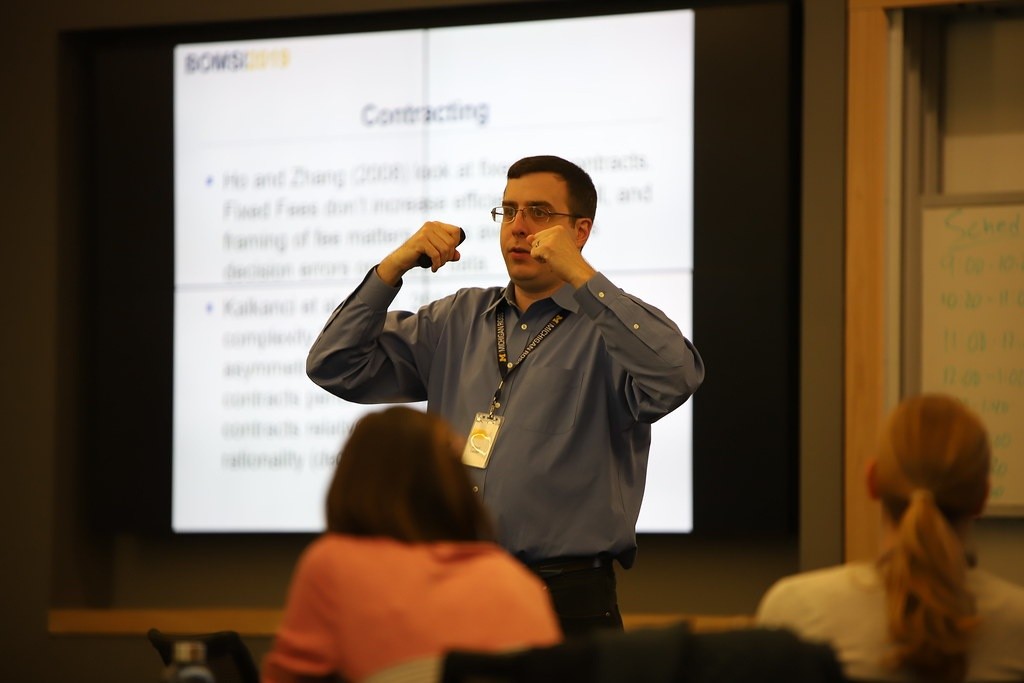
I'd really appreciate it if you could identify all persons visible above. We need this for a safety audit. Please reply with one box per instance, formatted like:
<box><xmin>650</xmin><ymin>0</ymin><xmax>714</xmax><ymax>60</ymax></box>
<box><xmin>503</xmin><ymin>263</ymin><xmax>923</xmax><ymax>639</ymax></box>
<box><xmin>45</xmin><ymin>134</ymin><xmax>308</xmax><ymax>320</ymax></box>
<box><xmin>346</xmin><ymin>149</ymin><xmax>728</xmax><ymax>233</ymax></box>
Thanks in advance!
<box><xmin>260</xmin><ymin>401</ymin><xmax>569</xmax><ymax>682</ymax></box>
<box><xmin>755</xmin><ymin>393</ymin><xmax>1023</xmax><ymax>683</ymax></box>
<box><xmin>303</xmin><ymin>154</ymin><xmax>706</xmax><ymax>641</ymax></box>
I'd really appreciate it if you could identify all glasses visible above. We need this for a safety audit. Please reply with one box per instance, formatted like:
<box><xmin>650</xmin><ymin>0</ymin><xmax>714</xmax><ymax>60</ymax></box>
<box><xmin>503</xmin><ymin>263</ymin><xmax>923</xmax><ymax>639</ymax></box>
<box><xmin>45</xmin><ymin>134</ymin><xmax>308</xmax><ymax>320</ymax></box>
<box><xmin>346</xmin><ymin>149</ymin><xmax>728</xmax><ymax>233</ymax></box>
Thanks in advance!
<box><xmin>492</xmin><ymin>207</ymin><xmax>582</xmax><ymax>225</ymax></box>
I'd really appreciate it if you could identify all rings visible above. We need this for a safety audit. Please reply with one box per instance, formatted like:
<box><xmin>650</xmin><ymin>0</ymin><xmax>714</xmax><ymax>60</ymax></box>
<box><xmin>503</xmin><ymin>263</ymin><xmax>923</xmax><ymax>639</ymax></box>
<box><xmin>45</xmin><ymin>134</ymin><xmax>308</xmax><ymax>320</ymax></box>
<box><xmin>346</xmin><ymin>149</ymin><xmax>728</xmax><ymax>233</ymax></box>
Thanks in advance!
<box><xmin>535</xmin><ymin>240</ymin><xmax>541</xmax><ymax>247</ymax></box>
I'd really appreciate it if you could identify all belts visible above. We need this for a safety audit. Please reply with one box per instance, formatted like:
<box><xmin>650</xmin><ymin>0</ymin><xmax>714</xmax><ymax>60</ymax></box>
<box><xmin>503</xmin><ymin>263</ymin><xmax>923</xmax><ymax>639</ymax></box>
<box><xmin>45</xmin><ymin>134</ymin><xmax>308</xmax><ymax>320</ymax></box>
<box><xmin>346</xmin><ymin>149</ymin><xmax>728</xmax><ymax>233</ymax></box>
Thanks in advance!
<box><xmin>525</xmin><ymin>558</ymin><xmax>614</xmax><ymax>578</ymax></box>
